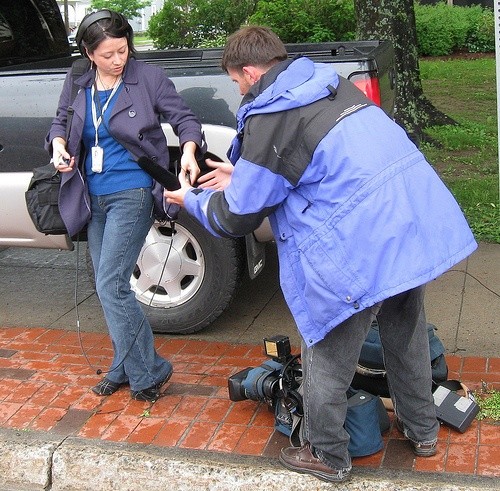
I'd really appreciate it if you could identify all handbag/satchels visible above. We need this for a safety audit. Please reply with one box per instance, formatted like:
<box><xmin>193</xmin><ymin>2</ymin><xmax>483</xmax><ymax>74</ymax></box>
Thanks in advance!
<box><xmin>25</xmin><ymin>162</ymin><xmax>68</xmax><ymax>235</ymax></box>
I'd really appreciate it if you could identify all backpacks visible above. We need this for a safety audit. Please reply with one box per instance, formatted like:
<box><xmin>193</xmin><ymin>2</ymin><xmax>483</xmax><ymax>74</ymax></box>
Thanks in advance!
<box><xmin>351</xmin><ymin>317</ymin><xmax>448</xmax><ymax>399</ymax></box>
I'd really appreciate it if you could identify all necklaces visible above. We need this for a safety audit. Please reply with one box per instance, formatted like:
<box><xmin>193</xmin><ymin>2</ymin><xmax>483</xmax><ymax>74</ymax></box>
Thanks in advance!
<box><xmin>102</xmin><ymin>80</ymin><xmax>111</xmax><ymax>89</ymax></box>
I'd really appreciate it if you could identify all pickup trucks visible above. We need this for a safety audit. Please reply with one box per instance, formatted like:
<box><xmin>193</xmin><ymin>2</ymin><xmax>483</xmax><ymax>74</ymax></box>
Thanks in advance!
<box><xmin>0</xmin><ymin>0</ymin><xmax>398</xmax><ymax>334</ymax></box>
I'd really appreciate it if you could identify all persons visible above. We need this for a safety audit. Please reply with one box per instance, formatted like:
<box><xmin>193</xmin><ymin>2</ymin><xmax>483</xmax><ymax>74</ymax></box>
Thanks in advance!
<box><xmin>42</xmin><ymin>9</ymin><xmax>207</xmax><ymax>401</ymax></box>
<box><xmin>164</xmin><ymin>25</ymin><xmax>479</xmax><ymax>483</ymax></box>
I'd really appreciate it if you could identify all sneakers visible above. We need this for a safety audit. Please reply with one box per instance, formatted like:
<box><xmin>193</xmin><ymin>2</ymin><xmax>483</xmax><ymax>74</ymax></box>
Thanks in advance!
<box><xmin>395</xmin><ymin>416</ymin><xmax>437</xmax><ymax>457</ymax></box>
<box><xmin>279</xmin><ymin>441</ymin><xmax>350</xmax><ymax>482</ymax></box>
<box><xmin>92</xmin><ymin>377</ymin><xmax>120</xmax><ymax>397</ymax></box>
<box><xmin>131</xmin><ymin>368</ymin><xmax>173</xmax><ymax>401</ymax></box>
